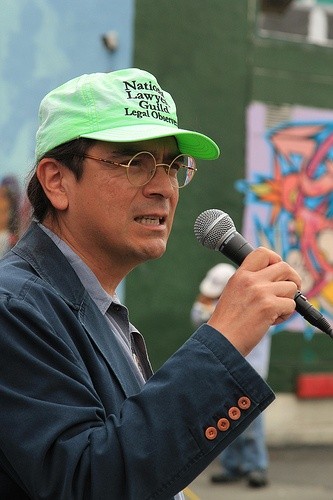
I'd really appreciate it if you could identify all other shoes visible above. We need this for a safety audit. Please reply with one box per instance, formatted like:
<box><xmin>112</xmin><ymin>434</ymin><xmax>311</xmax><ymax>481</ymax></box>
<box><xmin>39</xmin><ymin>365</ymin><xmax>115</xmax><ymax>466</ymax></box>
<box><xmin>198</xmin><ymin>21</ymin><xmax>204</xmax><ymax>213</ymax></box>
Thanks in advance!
<box><xmin>211</xmin><ymin>470</ymin><xmax>245</xmax><ymax>484</ymax></box>
<box><xmin>247</xmin><ymin>468</ymin><xmax>267</xmax><ymax>487</ymax></box>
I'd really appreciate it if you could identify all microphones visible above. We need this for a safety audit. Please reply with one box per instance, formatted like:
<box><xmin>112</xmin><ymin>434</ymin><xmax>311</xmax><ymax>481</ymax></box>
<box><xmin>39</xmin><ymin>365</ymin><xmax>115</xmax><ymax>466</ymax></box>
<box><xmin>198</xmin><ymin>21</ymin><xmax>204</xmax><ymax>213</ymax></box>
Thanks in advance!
<box><xmin>194</xmin><ymin>208</ymin><xmax>333</xmax><ymax>339</ymax></box>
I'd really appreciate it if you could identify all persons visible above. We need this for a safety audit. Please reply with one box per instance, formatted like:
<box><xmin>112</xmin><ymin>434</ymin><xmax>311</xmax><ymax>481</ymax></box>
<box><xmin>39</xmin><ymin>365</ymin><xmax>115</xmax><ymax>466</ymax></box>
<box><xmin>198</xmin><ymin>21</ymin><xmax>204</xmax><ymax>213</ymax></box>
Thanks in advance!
<box><xmin>0</xmin><ymin>68</ymin><xmax>302</xmax><ymax>500</ymax></box>
<box><xmin>0</xmin><ymin>176</ymin><xmax>23</xmax><ymax>259</ymax></box>
<box><xmin>191</xmin><ymin>264</ymin><xmax>271</xmax><ymax>486</ymax></box>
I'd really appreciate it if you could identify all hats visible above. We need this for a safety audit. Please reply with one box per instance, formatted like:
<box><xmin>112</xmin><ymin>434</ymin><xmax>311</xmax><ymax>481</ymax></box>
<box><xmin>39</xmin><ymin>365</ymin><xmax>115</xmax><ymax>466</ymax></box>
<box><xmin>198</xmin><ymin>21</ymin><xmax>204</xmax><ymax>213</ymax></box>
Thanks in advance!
<box><xmin>33</xmin><ymin>67</ymin><xmax>221</xmax><ymax>160</ymax></box>
<box><xmin>198</xmin><ymin>263</ymin><xmax>237</xmax><ymax>299</ymax></box>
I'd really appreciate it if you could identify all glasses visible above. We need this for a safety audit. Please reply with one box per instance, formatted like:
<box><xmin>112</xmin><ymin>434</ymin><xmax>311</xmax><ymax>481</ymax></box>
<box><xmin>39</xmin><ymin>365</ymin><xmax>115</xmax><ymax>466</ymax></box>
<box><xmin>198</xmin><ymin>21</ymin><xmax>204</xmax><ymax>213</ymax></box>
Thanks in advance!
<box><xmin>63</xmin><ymin>150</ymin><xmax>198</xmax><ymax>188</ymax></box>
